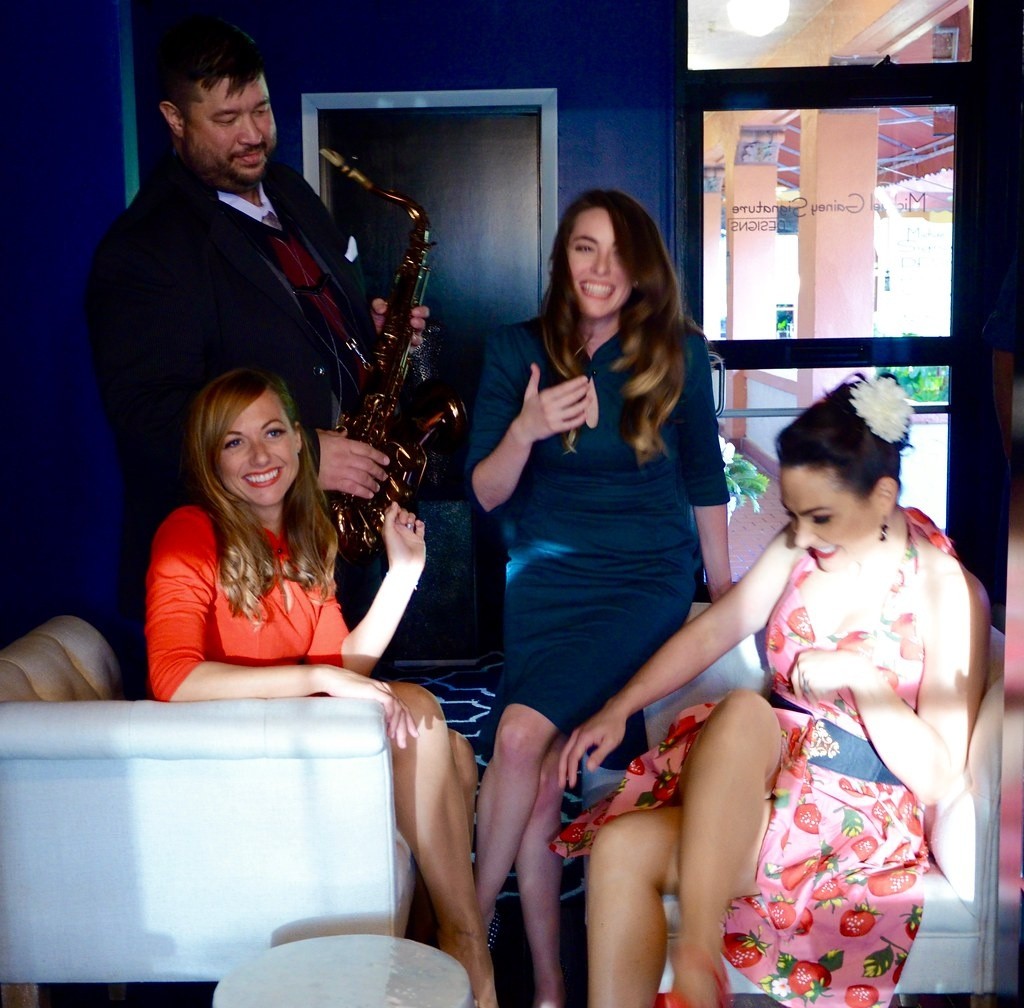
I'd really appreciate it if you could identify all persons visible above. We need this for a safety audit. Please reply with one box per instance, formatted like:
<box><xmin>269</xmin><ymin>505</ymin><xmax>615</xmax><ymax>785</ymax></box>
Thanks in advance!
<box><xmin>463</xmin><ymin>189</ymin><xmax>734</xmax><ymax>1008</ymax></box>
<box><xmin>143</xmin><ymin>367</ymin><xmax>500</xmax><ymax>1008</ymax></box>
<box><xmin>85</xmin><ymin>12</ymin><xmax>432</xmax><ymax>625</ymax></box>
<box><xmin>557</xmin><ymin>371</ymin><xmax>991</xmax><ymax>1008</ymax></box>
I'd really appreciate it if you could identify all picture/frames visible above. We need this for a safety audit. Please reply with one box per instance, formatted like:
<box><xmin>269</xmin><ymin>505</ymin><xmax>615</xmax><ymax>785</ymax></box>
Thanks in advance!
<box><xmin>301</xmin><ymin>88</ymin><xmax>555</xmax><ymax>338</ymax></box>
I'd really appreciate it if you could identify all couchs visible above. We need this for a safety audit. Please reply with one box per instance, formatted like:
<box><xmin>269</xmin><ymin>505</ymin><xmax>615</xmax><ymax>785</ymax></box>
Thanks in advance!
<box><xmin>657</xmin><ymin>602</ymin><xmax>1003</xmax><ymax>994</ymax></box>
<box><xmin>0</xmin><ymin>616</ymin><xmax>414</xmax><ymax>984</ymax></box>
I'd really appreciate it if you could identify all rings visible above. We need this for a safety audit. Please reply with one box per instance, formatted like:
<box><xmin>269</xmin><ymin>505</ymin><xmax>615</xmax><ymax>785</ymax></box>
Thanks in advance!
<box><xmin>406</xmin><ymin>523</ymin><xmax>413</xmax><ymax>528</ymax></box>
<box><xmin>804</xmin><ymin>686</ymin><xmax>810</xmax><ymax>692</ymax></box>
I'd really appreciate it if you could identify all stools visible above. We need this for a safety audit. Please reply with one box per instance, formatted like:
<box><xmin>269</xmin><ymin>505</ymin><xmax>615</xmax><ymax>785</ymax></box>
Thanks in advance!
<box><xmin>212</xmin><ymin>934</ymin><xmax>479</xmax><ymax>1008</ymax></box>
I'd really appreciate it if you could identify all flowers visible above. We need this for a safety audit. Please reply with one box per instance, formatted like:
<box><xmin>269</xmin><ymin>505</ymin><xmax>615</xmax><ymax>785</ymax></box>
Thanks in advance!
<box><xmin>718</xmin><ymin>434</ymin><xmax>769</xmax><ymax>513</ymax></box>
<box><xmin>849</xmin><ymin>377</ymin><xmax>912</xmax><ymax>444</ymax></box>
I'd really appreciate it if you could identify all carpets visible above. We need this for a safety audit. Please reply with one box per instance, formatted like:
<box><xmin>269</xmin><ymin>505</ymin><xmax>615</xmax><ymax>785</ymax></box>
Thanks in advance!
<box><xmin>389</xmin><ymin>652</ymin><xmax>586</xmax><ymax>900</ymax></box>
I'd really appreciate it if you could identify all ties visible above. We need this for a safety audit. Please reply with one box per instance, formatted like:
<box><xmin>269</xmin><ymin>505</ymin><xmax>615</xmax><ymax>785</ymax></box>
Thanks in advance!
<box><xmin>262</xmin><ymin>210</ymin><xmax>282</xmax><ymax>229</ymax></box>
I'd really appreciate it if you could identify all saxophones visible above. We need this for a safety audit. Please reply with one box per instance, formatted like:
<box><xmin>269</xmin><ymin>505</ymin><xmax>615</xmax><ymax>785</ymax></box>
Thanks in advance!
<box><xmin>319</xmin><ymin>145</ymin><xmax>475</xmax><ymax>570</ymax></box>
